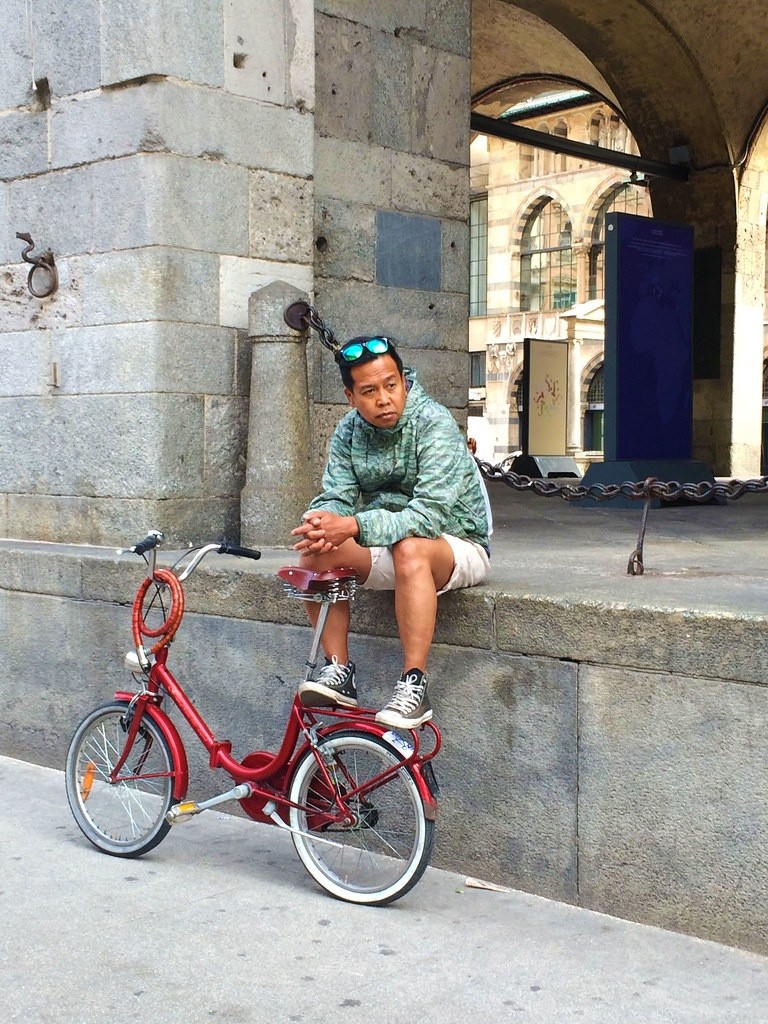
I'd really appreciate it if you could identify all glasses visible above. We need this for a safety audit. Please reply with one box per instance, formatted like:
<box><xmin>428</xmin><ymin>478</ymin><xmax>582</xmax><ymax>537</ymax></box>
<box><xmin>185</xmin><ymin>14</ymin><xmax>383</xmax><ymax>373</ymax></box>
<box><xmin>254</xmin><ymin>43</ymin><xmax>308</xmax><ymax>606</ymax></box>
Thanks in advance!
<box><xmin>335</xmin><ymin>336</ymin><xmax>394</xmax><ymax>360</ymax></box>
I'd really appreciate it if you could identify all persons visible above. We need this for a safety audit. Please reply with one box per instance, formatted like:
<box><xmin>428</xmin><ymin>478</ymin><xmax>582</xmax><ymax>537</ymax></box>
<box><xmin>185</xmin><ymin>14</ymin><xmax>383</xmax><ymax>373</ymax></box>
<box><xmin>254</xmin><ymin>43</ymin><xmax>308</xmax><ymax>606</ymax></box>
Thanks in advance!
<box><xmin>291</xmin><ymin>335</ymin><xmax>493</xmax><ymax>727</ymax></box>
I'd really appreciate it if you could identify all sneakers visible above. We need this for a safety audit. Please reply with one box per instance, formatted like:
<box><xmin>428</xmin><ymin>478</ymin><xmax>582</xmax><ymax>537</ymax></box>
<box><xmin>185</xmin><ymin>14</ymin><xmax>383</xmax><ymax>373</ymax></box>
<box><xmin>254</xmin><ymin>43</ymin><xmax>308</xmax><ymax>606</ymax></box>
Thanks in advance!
<box><xmin>373</xmin><ymin>670</ymin><xmax>434</xmax><ymax>727</ymax></box>
<box><xmin>297</xmin><ymin>654</ymin><xmax>359</xmax><ymax>710</ymax></box>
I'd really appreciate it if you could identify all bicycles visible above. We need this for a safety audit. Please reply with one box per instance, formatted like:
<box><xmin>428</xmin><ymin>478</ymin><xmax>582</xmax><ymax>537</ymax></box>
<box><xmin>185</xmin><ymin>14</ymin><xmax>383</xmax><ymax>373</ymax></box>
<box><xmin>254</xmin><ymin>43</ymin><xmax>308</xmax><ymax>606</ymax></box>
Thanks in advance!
<box><xmin>65</xmin><ymin>529</ymin><xmax>442</xmax><ymax>907</ymax></box>
